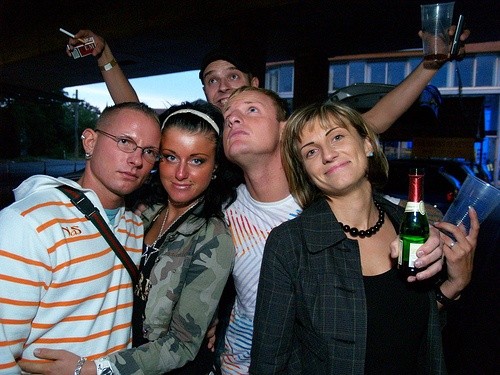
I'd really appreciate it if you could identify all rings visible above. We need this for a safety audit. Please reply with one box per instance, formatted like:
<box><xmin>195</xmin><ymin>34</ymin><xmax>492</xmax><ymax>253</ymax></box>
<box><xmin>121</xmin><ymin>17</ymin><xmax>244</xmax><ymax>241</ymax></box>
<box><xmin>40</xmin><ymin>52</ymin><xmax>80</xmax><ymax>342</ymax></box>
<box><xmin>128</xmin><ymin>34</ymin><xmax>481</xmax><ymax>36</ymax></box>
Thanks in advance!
<box><xmin>449</xmin><ymin>241</ymin><xmax>457</xmax><ymax>248</ymax></box>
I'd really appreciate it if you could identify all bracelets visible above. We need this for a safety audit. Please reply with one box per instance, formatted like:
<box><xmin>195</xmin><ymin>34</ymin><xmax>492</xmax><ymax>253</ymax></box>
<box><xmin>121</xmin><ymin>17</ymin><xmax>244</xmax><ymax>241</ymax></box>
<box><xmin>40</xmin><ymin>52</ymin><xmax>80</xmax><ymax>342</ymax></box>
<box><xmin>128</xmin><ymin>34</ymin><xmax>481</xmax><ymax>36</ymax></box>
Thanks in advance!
<box><xmin>432</xmin><ymin>280</ymin><xmax>463</xmax><ymax>305</ymax></box>
<box><xmin>98</xmin><ymin>57</ymin><xmax>116</xmax><ymax>72</ymax></box>
<box><xmin>74</xmin><ymin>356</ymin><xmax>86</xmax><ymax>375</ymax></box>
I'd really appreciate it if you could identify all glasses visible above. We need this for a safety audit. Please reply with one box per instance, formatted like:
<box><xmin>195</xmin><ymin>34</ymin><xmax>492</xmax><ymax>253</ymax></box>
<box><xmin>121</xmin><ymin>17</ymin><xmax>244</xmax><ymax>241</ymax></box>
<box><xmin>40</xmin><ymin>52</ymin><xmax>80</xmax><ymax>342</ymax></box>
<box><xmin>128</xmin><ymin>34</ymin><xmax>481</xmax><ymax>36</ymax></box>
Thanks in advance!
<box><xmin>94</xmin><ymin>128</ymin><xmax>163</xmax><ymax>163</ymax></box>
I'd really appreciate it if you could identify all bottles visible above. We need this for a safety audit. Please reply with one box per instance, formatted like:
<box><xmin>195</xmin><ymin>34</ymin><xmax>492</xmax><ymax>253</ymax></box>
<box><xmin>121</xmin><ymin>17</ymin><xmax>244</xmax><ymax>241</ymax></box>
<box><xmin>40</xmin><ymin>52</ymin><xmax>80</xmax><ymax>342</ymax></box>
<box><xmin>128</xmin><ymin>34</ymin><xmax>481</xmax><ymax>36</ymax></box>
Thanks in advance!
<box><xmin>398</xmin><ymin>167</ymin><xmax>429</xmax><ymax>276</ymax></box>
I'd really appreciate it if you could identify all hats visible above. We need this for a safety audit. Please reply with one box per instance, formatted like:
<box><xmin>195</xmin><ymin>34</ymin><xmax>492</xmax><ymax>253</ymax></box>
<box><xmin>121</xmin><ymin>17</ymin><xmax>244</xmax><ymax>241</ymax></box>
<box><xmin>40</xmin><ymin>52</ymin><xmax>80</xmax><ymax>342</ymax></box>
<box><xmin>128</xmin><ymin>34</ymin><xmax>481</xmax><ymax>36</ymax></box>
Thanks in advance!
<box><xmin>199</xmin><ymin>46</ymin><xmax>253</xmax><ymax>73</ymax></box>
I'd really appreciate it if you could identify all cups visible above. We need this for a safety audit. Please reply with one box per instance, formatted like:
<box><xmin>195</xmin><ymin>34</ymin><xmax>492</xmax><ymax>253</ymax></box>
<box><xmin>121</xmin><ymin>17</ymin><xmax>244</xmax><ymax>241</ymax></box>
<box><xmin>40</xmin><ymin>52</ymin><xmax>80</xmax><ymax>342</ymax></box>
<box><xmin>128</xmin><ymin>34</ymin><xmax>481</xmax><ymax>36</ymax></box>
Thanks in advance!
<box><xmin>419</xmin><ymin>1</ymin><xmax>456</xmax><ymax>62</ymax></box>
<box><xmin>439</xmin><ymin>173</ymin><xmax>500</xmax><ymax>241</ymax></box>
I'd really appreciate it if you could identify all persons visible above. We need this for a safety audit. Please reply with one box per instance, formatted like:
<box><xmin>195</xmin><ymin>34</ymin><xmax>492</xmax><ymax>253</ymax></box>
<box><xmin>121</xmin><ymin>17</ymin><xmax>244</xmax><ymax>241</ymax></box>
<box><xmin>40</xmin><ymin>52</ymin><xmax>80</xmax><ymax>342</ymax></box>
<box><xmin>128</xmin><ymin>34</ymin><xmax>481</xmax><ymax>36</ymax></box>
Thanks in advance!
<box><xmin>0</xmin><ymin>86</ymin><xmax>500</xmax><ymax>375</ymax></box>
<box><xmin>65</xmin><ymin>24</ymin><xmax>470</xmax><ymax>187</ymax></box>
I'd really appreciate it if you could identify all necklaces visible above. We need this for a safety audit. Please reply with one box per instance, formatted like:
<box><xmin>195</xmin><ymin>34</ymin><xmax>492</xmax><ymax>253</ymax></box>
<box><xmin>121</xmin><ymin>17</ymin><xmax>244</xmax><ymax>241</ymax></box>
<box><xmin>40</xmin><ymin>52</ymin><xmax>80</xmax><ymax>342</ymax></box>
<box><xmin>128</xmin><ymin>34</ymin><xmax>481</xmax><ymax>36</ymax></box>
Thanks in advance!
<box><xmin>141</xmin><ymin>199</ymin><xmax>202</xmax><ymax>266</ymax></box>
<box><xmin>340</xmin><ymin>199</ymin><xmax>384</xmax><ymax>237</ymax></box>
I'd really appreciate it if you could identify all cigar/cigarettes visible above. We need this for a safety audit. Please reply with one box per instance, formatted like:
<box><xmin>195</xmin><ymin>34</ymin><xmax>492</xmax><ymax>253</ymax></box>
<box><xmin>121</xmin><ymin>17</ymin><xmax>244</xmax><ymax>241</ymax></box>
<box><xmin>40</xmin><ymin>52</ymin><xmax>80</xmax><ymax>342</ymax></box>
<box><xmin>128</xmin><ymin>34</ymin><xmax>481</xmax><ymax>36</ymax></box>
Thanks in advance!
<box><xmin>59</xmin><ymin>28</ymin><xmax>84</xmax><ymax>43</ymax></box>
